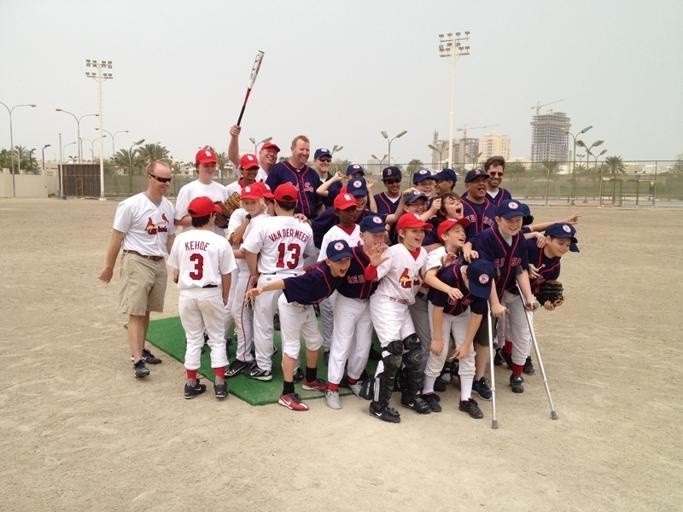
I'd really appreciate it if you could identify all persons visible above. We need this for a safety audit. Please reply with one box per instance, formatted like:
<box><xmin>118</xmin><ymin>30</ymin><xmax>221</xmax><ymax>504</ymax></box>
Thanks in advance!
<box><xmin>462</xmin><ymin>199</ymin><xmax>540</xmax><ymax>401</ymax></box>
<box><xmin>246</xmin><ymin>240</ymin><xmax>353</xmax><ymax>411</ymax></box>
<box><xmin>168</xmin><ymin>196</ymin><xmax>239</xmax><ymax>399</ymax></box>
<box><xmin>296</xmin><ymin>215</ymin><xmax>442</xmax><ymax>410</ymax></box>
<box><xmin>419</xmin><ymin>260</ymin><xmax>494</xmax><ymax>419</ymax></box>
<box><xmin>174</xmin><ymin>127</ymin><xmax>582</xmax><ymax>393</ymax></box>
<box><xmin>98</xmin><ymin>159</ymin><xmax>176</xmax><ymax>378</ymax></box>
<box><xmin>364</xmin><ymin>212</ymin><xmax>457</xmax><ymax>422</ymax></box>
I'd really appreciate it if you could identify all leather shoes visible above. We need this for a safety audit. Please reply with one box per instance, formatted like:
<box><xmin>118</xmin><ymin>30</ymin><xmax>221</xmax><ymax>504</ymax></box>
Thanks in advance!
<box><xmin>203</xmin><ymin>284</ymin><xmax>218</xmax><ymax>288</ymax></box>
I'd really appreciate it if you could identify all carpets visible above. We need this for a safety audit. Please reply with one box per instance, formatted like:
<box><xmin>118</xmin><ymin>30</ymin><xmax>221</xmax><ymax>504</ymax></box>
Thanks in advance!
<box><xmin>120</xmin><ymin>312</ymin><xmax>383</xmax><ymax>410</ymax></box>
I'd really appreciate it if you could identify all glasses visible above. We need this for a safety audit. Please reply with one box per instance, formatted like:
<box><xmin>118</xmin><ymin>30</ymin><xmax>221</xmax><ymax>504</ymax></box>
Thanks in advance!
<box><xmin>487</xmin><ymin>171</ymin><xmax>504</xmax><ymax>176</ymax></box>
<box><xmin>146</xmin><ymin>173</ymin><xmax>172</xmax><ymax>183</ymax></box>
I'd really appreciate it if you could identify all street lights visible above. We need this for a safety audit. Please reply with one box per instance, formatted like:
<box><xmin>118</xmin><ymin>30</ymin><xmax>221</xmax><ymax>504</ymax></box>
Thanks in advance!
<box><xmin>378</xmin><ymin>127</ymin><xmax>407</xmax><ymax>168</ymax></box>
<box><xmin>82</xmin><ymin>56</ymin><xmax>116</xmax><ymax>202</ymax></box>
<box><xmin>563</xmin><ymin>125</ymin><xmax>607</xmax><ymax>207</ymax></box>
<box><xmin>0</xmin><ymin>102</ymin><xmax>135</xmax><ymax>200</ymax></box>
<box><xmin>248</xmin><ymin>135</ymin><xmax>272</xmax><ymax>157</ymax></box>
<box><xmin>369</xmin><ymin>153</ymin><xmax>389</xmax><ymax>174</ymax></box>
<box><xmin>435</xmin><ymin>30</ymin><xmax>470</xmax><ymax>170</ymax></box>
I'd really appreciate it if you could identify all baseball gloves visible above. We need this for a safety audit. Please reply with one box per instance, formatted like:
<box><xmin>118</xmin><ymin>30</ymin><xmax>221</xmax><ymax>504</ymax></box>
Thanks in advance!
<box><xmin>216</xmin><ymin>192</ymin><xmax>242</xmax><ymax>229</ymax></box>
<box><xmin>537</xmin><ymin>278</ymin><xmax>562</xmax><ymax>308</ymax></box>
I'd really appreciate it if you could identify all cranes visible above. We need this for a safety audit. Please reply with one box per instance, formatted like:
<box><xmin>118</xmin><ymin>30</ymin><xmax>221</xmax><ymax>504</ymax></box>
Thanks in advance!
<box><xmin>528</xmin><ymin>96</ymin><xmax>568</xmax><ymax>114</ymax></box>
<box><xmin>457</xmin><ymin>120</ymin><xmax>501</xmax><ymax>139</ymax></box>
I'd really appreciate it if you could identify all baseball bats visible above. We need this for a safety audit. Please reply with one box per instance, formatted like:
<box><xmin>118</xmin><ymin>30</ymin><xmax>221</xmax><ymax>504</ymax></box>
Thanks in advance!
<box><xmin>236</xmin><ymin>51</ymin><xmax>264</xmax><ymax>126</ymax></box>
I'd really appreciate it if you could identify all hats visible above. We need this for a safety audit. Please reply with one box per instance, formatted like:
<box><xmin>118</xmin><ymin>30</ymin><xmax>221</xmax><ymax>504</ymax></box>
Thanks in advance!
<box><xmin>261</xmin><ymin>142</ymin><xmax>280</xmax><ymax>152</ymax></box>
<box><xmin>521</xmin><ymin>204</ymin><xmax>534</xmax><ymax>225</ymax></box>
<box><xmin>187</xmin><ymin>196</ymin><xmax>223</xmax><ymax>217</ymax></box>
<box><xmin>496</xmin><ymin>199</ymin><xmax>529</xmax><ymax>218</ymax></box>
<box><xmin>195</xmin><ymin>146</ymin><xmax>217</xmax><ymax>164</ymax></box>
<box><xmin>359</xmin><ymin>215</ymin><xmax>385</xmax><ymax>234</ymax></box>
<box><xmin>326</xmin><ymin>239</ymin><xmax>353</xmax><ymax>260</ymax></box>
<box><xmin>404</xmin><ymin>189</ymin><xmax>429</xmax><ymax>203</ymax></box>
<box><xmin>313</xmin><ymin>147</ymin><xmax>490</xmax><ymax>183</ymax></box>
<box><xmin>544</xmin><ymin>223</ymin><xmax>580</xmax><ymax>253</ymax></box>
<box><xmin>469</xmin><ymin>258</ymin><xmax>495</xmax><ymax>299</ymax></box>
<box><xmin>239</xmin><ymin>154</ymin><xmax>260</xmax><ymax>170</ymax></box>
<box><xmin>347</xmin><ymin>177</ymin><xmax>368</xmax><ymax>196</ymax></box>
<box><xmin>395</xmin><ymin>213</ymin><xmax>434</xmax><ymax>232</ymax></box>
<box><xmin>240</xmin><ymin>182</ymin><xmax>299</xmax><ymax>202</ymax></box>
<box><xmin>333</xmin><ymin>193</ymin><xmax>358</xmax><ymax>211</ymax></box>
<box><xmin>436</xmin><ymin>218</ymin><xmax>470</xmax><ymax>241</ymax></box>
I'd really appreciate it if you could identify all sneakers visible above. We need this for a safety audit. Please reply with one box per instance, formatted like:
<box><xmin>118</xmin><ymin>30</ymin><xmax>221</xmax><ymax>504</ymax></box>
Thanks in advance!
<box><xmin>184</xmin><ymin>379</ymin><xmax>206</xmax><ymax>400</ymax></box>
<box><xmin>434</xmin><ymin>361</ymin><xmax>460</xmax><ymax>392</ymax></box>
<box><xmin>214</xmin><ymin>381</ymin><xmax>229</xmax><ymax>398</ymax></box>
<box><xmin>133</xmin><ymin>360</ymin><xmax>150</xmax><ymax>379</ymax></box>
<box><xmin>278</xmin><ymin>393</ymin><xmax>309</xmax><ymax>412</ymax></box>
<box><xmin>131</xmin><ymin>350</ymin><xmax>161</xmax><ymax>364</ymax></box>
<box><xmin>494</xmin><ymin>347</ymin><xmax>535</xmax><ymax>374</ymax></box>
<box><xmin>472</xmin><ymin>378</ymin><xmax>493</xmax><ymax>401</ymax></box>
<box><xmin>243</xmin><ymin>366</ymin><xmax>273</xmax><ymax>381</ymax></box>
<box><xmin>293</xmin><ymin>365</ymin><xmax>365</xmax><ymax>409</ymax></box>
<box><xmin>224</xmin><ymin>356</ymin><xmax>255</xmax><ymax>377</ymax></box>
<box><xmin>370</xmin><ymin>393</ymin><xmax>483</xmax><ymax>423</ymax></box>
<box><xmin>509</xmin><ymin>375</ymin><xmax>524</xmax><ymax>394</ymax></box>
<box><xmin>274</xmin><ymin>314</ymin><xmax>280</xmax><ymax>331</ymax></box>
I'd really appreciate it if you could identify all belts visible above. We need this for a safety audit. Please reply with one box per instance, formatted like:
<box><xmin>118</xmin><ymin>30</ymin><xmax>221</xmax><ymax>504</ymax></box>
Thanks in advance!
<box><xmin>122</xmin><ymin>250</ymin><xmax>164</xmax><ymax>261</ymax></box>
